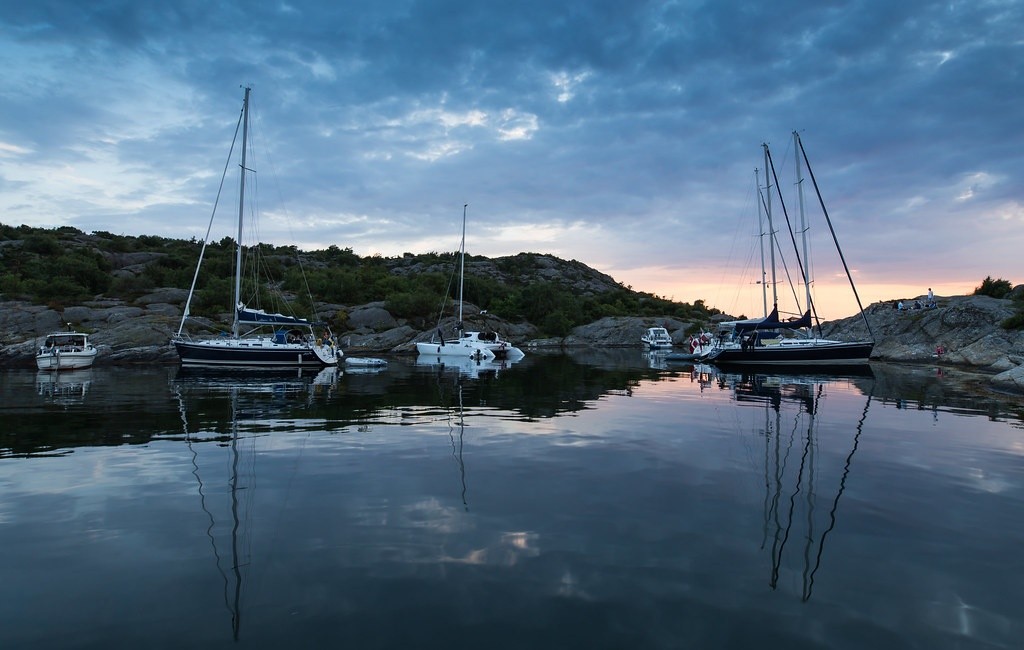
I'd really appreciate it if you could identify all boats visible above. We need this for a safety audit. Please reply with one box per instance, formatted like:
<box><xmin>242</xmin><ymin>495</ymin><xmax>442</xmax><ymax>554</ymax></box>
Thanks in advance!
<box><xmin>34</xmin><ymin>331</ymin><xmax>98</xmax><ymax>370</ymax></box>
<box><xmin>343</xmin><ymin>353</ymin><xmax>387</xmax><ymax>369</ymax></box>
<box><xmin>641</xmin><ymin>327</ymin><xmax>674</xmax><ymax>347</ymax></box>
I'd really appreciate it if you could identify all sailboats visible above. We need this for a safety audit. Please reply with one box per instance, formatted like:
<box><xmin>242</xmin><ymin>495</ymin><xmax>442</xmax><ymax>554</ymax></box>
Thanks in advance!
<box><xmin>416</xmin><ymin>205</ymin><xmax>526</xmax><ymax>372</ymax></box>
<box><xmin>159</xmin><ymin>84</ymin><xmax>347</xmax><ymax>368</ymax></box>
<box><xmin>704</xmin><ymin>128</ymin><xmax>877</xmax><ymax>373</ymax></box>
